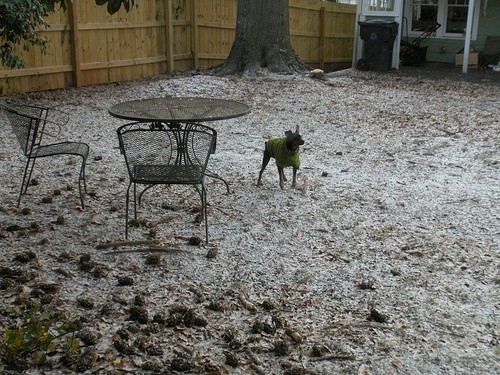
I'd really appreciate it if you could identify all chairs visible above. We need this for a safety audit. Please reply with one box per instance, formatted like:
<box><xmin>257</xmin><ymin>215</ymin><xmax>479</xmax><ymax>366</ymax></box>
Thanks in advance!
<box><xmin>116</xmin><ymin>119</ymin><xmax>218</xmax><ymax>244</ymax></box>
<box><xmin>0</xmin><ymin>97</ymin><xmax>90</xmax><ymax>210</ymax></box>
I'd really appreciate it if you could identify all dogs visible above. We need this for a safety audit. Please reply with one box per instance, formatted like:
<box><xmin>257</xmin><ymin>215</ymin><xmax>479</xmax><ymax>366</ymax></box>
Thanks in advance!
<box><xmin>257</xmin><ymin>124</ymin><xmax>305</xmax><ymax>190</ymax></box>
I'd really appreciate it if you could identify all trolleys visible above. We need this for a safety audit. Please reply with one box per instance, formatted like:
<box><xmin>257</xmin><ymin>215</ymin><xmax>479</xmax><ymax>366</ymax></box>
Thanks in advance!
<box><xmin>398</xmin><ymin>16</ymin><xmax>441</xmax><ymax>68</ymax></box>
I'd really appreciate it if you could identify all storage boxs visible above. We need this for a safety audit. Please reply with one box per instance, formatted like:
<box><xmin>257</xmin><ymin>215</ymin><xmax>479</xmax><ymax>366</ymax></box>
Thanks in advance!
<box><xmin>455</xmin><ymin>45</ymin><xmax>479</xmax><ymax>68</ymax></box>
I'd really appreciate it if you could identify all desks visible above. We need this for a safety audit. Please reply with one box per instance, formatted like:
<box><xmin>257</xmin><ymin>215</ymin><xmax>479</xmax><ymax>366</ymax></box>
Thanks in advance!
<box><xmin>108</xmin><ymin>97</ymin><xmax>251</xmax><ymax>209</ymax></box>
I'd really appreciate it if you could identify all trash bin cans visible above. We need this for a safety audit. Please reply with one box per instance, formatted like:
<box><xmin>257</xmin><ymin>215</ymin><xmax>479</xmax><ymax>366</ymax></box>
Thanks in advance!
<box><xmin>355</xmin><ymin>17</ymin><xmax>400</xmax><ymax>72</ymax></box>
